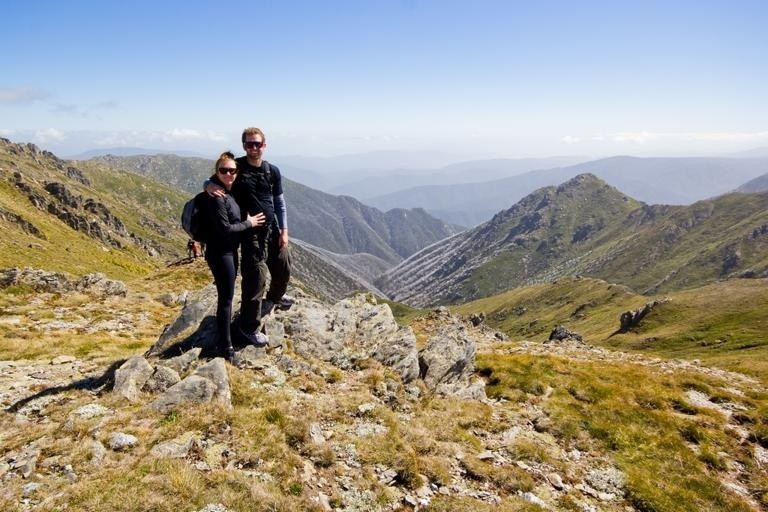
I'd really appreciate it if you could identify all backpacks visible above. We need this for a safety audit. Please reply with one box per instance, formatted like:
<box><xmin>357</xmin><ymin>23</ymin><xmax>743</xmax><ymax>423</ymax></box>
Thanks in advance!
<box><xmin>180</xmin><ymin>192</ymin><xmax>207</xmax><ymax>242</ymax></box>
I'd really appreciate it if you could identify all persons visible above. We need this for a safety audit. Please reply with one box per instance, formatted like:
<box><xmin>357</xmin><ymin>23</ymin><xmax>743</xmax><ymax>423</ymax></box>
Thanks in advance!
<box><xmin>197</xmin><ymin>150</ymin><xmax>267</xmax><ymax>362</ymax></box>
<box><xmin>202</xmin><ymin>127</ymin><xmax>297</xmax><ymax>347</ymax></box>
<box><xmin>187</xmin><ymin>239</ymin><xmax>194</xmax><ymax>259</ymax></box>
<box><xmin>190</xmin><ymin>241</ymin><xmax>201</xmax><ymax>257</ymax></box>
<box><xmin>199</xmin><ymin>242</ymin><xmax>207</xmax><ymax>257</ymax></box>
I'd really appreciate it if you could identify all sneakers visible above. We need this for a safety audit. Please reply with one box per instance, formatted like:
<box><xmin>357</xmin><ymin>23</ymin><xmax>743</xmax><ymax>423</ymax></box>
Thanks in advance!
<box><xmin>265</xmin><ymin>290</ymin><xmax>295</xmax><ymax>306</ymax></box>
<box><xmin>219</xmin><ymin>345</ymin><xmax>244</xmax><ymax>369</ymax></box>
<box><xmin>238</xmin><ymin>324</ymin><xmax>267</xmax><ymax>347</ymax></box>
